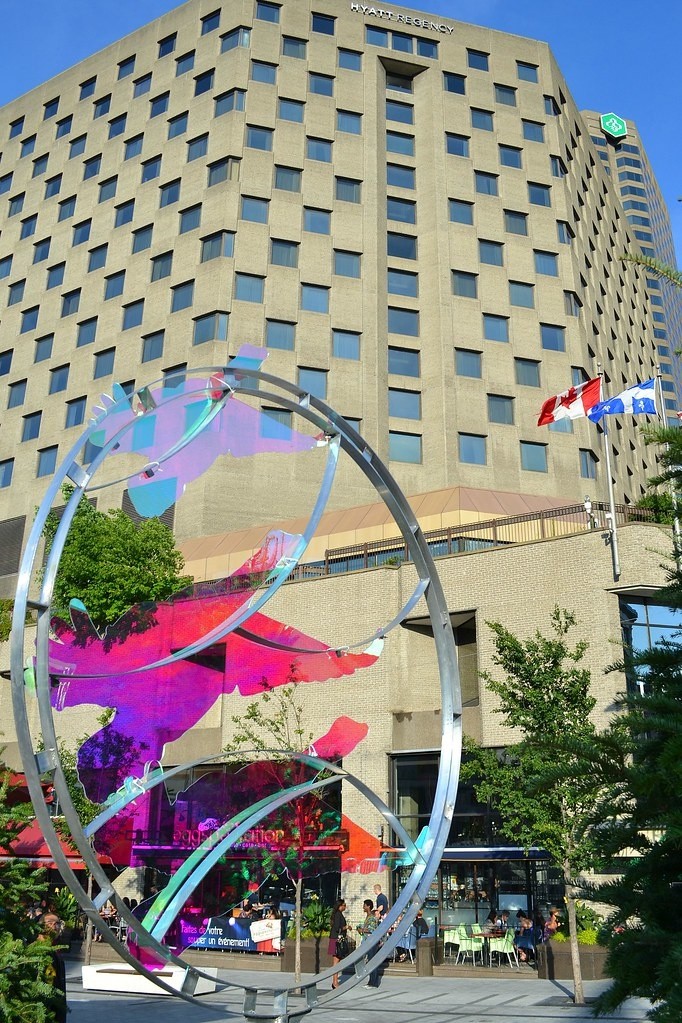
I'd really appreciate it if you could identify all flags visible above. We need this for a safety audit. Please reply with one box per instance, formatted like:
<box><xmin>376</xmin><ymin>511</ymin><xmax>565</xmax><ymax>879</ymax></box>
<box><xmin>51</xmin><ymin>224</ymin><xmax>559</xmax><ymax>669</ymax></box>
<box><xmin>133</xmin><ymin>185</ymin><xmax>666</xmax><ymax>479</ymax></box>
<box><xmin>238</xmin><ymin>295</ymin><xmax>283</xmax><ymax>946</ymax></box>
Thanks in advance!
<box><xmin>589</xmin><ymin>379</ymin><xmax>657</xmax><ymax>422</ymax></box>
<box><xmin>537</xmin><ymin>377</ymin><xmax>600</xmax><ymax>426</ymax></box>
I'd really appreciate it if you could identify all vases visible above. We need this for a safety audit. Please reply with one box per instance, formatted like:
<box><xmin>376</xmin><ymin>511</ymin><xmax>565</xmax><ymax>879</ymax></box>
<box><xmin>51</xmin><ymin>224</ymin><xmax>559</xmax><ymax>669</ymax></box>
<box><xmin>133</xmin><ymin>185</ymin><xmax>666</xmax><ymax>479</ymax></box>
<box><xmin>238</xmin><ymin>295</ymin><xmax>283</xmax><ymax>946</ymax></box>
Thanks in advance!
<box><xmin>281</xmin><ymin>934</ymin><xmax>356</xmax><ymax>973</ymax></box>
<box><xmin>536</xmin><ymin>941</ymin><xmax>611</xmax><ymax>980</ymax></box>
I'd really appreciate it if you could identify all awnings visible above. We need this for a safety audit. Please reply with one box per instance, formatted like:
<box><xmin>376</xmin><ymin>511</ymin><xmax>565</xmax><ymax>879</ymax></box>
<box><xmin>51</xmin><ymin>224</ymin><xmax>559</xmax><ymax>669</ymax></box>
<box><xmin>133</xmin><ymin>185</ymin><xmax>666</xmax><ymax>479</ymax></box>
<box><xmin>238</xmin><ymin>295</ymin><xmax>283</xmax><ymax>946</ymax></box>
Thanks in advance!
<box><xmin>0</xmin><ymin>816</ymin><xmax>134</xmax><ymax>869</ymax></box>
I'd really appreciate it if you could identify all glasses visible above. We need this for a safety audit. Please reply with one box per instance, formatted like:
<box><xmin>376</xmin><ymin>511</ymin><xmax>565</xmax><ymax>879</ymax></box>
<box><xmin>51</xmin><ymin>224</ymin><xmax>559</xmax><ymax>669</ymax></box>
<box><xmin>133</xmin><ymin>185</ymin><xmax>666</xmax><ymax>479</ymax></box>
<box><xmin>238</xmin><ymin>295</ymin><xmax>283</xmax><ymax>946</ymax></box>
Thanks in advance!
<box><xmin>341</xmin><ymin>904</ymin><xmax>346</xmax><ymax>907</ymax></box>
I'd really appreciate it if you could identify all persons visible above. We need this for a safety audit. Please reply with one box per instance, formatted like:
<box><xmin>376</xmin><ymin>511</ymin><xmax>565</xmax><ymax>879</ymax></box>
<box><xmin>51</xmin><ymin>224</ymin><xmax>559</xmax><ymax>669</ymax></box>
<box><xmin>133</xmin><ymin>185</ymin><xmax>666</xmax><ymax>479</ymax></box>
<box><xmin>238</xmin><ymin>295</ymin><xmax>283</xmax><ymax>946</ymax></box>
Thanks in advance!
<box><xmin>514</xmin><ymin>909</ymin><xmax>533</xmax><ymax>960</ymax></box>
<box><xmin>91</xmin><ymin>897</ymin><xmax>137</xmax><ymax>942</ymax></box>
<box><xmin>328</xmin><ymin>899</ymin><xmax>352</xmax><ymax>990</ymax></box>
<box><xmin>356</xmin><ymin>899</ymin><xmax>378</xmax><ymax>989</ymax></box>
<box><xmin>269</xmin><ymin>906</ymin><xmax>279</xmax><ymax>919</ymax></box>
<box><xmin>30</xmin><ymin>913</ymin><xmax>66</xmax><ymax>1023</ymax></box>
<box><xmin>544</xmin><ymin>906</ymin><xmax>560</xmax><ymax>939</ymax></box>
<box><xmin>150</xmin><ymin>885</ymin><xmax>160</xmax><ymax>893</ymax></box>
<box><xmin>395</xmin><ymin>908</ymin><xmax>429</xmax><ymax>962</ymax></box>
<box><xmin>16</xmin><ymin>905</ymin><xmax>56</xmax><ymax>922</ymax></box>
<box><xmin>373</xmin><ymin>885</ymin><xmax>391</xmax><ymax>947</ymax></box>
<box><xmin>482</xmin><ymin>909</ymin><xmax>510</xmax><ymax>934</ymax></box>
<box><xmin>238</xmin><ymin>904</ymin><xmax>254</xmax><ymax>919</ymax></box>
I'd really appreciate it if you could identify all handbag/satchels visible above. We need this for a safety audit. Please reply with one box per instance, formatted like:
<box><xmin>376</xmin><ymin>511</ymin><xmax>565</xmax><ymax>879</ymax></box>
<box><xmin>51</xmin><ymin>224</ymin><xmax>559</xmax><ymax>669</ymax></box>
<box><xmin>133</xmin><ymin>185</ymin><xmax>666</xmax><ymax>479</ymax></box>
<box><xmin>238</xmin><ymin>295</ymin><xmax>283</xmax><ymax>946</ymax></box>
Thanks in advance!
<box><xmin>335</xmin><ymin>927</ymin><xmax>349</xmax><ymax>955</ymax></box>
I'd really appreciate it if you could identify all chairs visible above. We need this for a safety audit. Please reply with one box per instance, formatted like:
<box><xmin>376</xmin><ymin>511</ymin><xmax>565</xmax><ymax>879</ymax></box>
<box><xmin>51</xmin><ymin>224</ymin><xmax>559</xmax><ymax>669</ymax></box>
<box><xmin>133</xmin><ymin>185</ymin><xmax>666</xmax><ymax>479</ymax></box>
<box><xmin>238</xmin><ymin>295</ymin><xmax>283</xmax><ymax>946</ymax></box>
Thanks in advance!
<box><xmin>393</xmin><ymin>924</ymin><xmax>439</xmax><ymax>965</ymax></box>
<box><xmin>443</xmin><ymin>923</ymin><xmax>541</xmax><ymax>969</ymax></box>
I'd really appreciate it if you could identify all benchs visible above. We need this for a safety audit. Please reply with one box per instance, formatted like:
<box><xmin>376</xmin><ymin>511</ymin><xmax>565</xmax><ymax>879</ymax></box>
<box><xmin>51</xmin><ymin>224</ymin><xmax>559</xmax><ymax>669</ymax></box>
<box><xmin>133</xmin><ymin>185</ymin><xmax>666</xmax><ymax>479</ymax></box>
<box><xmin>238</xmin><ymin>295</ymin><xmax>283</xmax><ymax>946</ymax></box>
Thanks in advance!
<box><xmin>469</xmin><ymin>933</ymin><xmax>504</xmax><ymax>968</ymax></box>
<box><xmin>494</xmin><ymin>930</ymin><xmax>521</xmax><ymax>935</ymax></box>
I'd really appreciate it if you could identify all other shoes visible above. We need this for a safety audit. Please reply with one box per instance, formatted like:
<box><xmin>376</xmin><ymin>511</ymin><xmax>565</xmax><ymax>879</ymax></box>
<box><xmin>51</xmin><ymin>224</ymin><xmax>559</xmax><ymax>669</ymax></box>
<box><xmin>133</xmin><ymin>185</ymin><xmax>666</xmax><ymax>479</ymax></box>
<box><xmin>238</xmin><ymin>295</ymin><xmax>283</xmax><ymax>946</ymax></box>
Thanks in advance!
<box><xmin>398</xmin><ymin>955</ymin><xmax>406</xmax><ymax>962</ymax></box>
<box><xmin>332</xmin><ymin>983</ymin><xmax>336</xmax><ymax>989</ymax></box>
<box><xmin>362</xmin><ymin>985</ymin><xmax>376</xmax><ymax>989</ymax></box>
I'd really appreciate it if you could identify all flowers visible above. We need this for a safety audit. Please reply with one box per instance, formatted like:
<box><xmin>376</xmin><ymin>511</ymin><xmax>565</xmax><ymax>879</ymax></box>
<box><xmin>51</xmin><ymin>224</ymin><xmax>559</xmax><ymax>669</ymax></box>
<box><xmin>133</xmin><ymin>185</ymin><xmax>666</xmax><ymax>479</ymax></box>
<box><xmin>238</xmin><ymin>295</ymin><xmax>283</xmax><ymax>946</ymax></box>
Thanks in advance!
<box><xmin>549</xmin><ymin>894</ymin><xmax>605</xmax><ymax>946</ymax></box>
<box><xmin>287</xmin><ymin>894</ymin><xmax>350</xmax><ymax>940</ymax></box>
